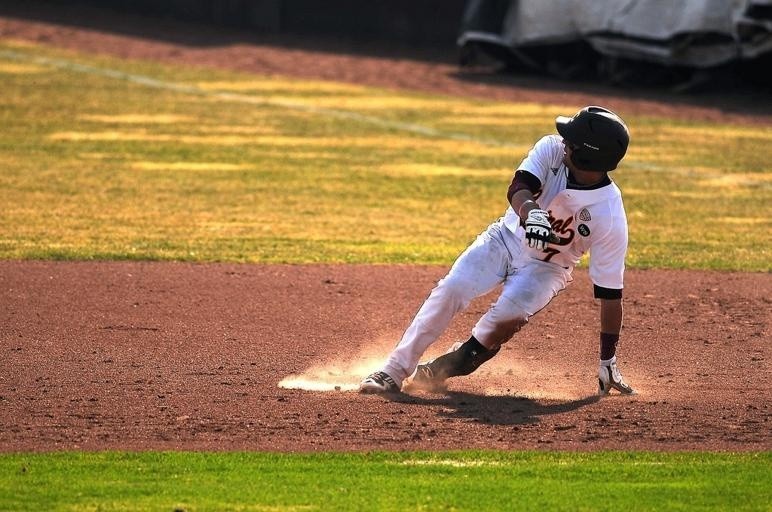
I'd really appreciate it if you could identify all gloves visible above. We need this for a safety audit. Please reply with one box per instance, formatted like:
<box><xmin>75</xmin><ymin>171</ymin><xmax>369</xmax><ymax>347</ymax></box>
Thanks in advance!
<box><xmin>522</xmin><ymin>208</ymin><xmax>561</xmax><ymax>251</ymax></box>
<box><xmin>597</xmin><ymin>355</ymin><xmax>638</xmax><ymax>396</ymax></box>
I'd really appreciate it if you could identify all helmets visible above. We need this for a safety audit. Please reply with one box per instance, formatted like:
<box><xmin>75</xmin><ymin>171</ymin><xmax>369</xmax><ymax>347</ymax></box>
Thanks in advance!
<box><xmin>555</xmin><ymin>106</ymin><xmax>630</xmax><ymax>171</ymax></box>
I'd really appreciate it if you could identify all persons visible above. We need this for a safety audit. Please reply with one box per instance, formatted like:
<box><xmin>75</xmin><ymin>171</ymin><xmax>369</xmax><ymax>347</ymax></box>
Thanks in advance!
<box><xmin>361</xmin><ymin>106</ymin><xmax>639</xmax><ymax>397</ymax></box>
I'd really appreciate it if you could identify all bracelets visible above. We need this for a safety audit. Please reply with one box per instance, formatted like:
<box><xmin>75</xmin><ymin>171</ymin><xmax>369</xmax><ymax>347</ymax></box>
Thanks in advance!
<box><xmin>598</xmin><ymin>332</ymin><xmax>624</xmax><ymax>361</ymax></box>
<box><xmin>518</xmin><ymin>198</ymin><xmax>539</xmax><ymax>218</ymax></box>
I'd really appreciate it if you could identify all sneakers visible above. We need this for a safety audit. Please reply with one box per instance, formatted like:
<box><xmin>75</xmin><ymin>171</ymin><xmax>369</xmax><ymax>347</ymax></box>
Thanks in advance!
<box><xmin>403</xmin><ymin>362</ymin><xmax>444</xmax><ymax>390</ymax></box>
<box><xmin>360</xmin><ymin>370</ymin><xmax>401</xmax><ymax>393</ymax></box>
